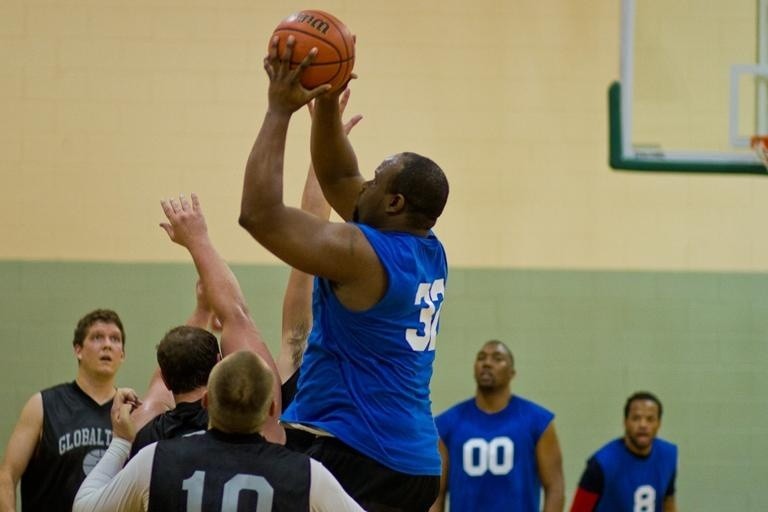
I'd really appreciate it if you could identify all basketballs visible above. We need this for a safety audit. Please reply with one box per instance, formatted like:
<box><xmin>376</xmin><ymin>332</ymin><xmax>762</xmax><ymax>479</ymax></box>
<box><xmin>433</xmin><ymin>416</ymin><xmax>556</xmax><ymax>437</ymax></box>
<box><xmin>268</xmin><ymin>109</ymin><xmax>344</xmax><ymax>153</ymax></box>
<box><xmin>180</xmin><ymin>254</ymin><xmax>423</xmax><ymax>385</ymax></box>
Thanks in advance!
<box><xmin>268</xmin><ymin>9</ymin><xmax>354</xmax><ymax>93</ymax></box>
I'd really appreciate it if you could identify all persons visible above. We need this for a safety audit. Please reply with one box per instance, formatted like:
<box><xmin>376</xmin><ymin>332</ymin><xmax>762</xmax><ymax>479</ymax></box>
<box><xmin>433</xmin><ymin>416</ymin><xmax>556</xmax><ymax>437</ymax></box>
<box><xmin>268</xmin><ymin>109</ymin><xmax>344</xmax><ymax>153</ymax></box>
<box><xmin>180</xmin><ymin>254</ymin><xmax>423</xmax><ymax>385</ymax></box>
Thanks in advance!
<box><xmin>569</xmin><ymin>391</ymin><xmax>679</xmax><ymax>512</ymax></box>
<box><xmin>70</xmin><ymin>83</ymin><xmax>365</xmax><ymax>512</ymax></box>
<box><xmin>427</xmin><ymin>341</ymin><xmax>565</xmax><ymax>512</ymax></box>
<box><xmin>236</xmin><ymin>36</ymin><xmax>449</xmax><ymax>511</ymax></box>
<box><xmin>2</xmin><ymin>306</ymin><xmax>126</xmax><ymax>512</ymax></box>
